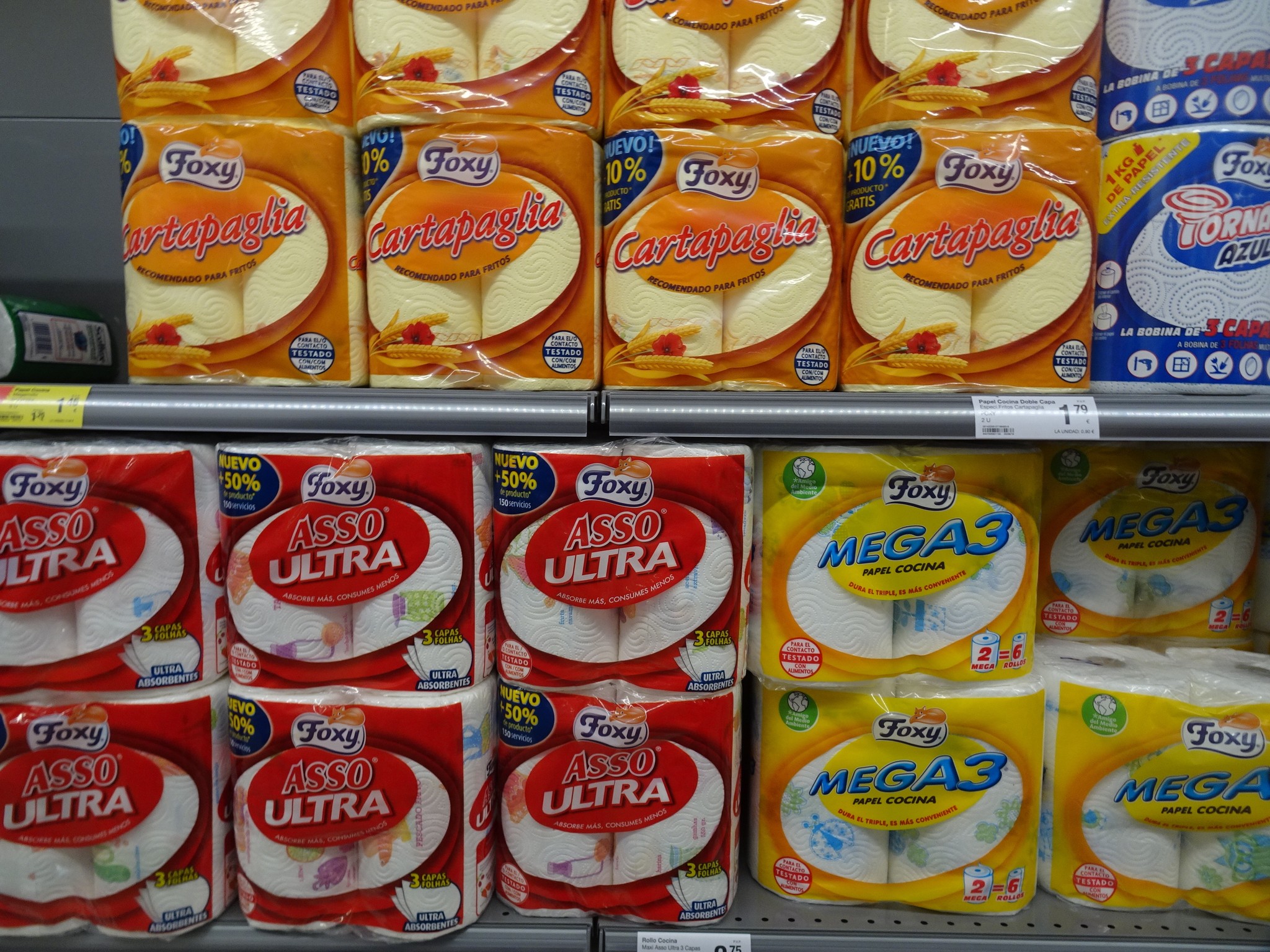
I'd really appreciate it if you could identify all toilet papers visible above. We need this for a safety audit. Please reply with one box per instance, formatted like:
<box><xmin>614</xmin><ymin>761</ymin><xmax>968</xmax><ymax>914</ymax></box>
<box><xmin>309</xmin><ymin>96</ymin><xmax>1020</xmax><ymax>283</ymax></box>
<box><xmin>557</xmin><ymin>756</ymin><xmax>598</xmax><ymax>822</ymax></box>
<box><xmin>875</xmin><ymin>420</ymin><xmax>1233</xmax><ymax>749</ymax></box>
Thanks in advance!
<box><xmin>0</xmin><ymin>0</ymin><xmax>1269</xmax><ymax>952</ymax></box>
